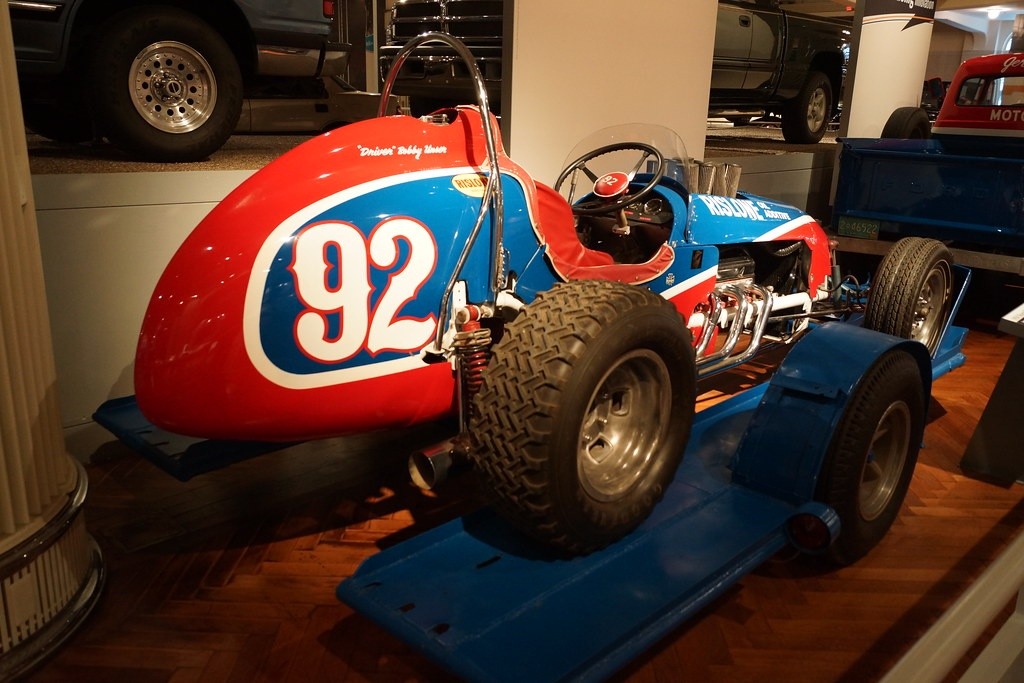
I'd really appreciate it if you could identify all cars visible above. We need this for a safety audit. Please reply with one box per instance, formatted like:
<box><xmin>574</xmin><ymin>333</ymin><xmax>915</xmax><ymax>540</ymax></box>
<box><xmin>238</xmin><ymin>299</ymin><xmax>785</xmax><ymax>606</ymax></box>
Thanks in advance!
<box><xmin>8</xmin><ymin>0</ymin><xmax>846</xmax><ymax>162</ymax></box>
